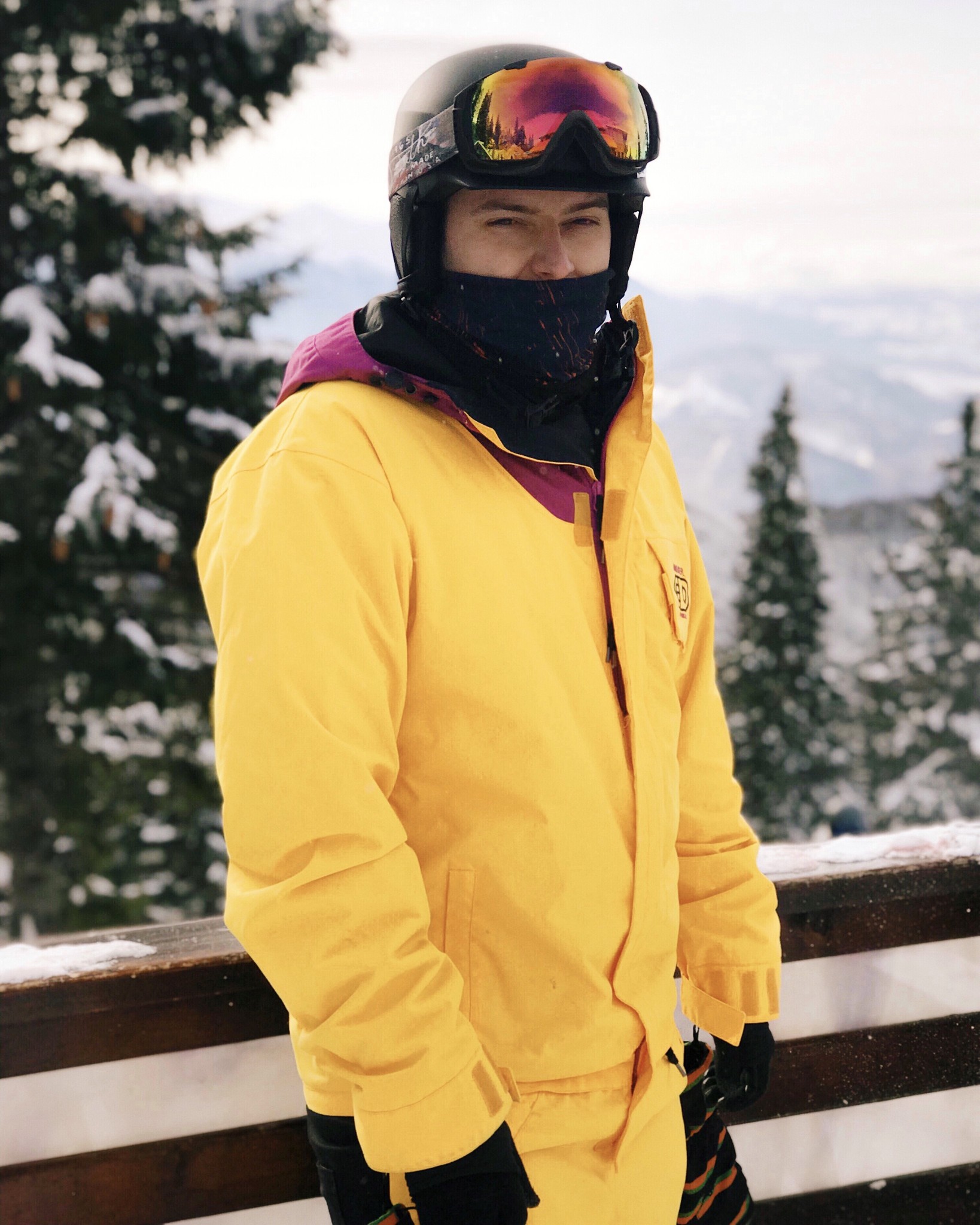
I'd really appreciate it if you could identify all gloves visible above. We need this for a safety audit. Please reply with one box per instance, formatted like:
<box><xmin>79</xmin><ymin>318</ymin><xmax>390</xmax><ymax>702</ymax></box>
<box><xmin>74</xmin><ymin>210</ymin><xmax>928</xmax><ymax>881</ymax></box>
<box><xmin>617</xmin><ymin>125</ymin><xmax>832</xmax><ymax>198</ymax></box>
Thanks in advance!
<box><xmin>712</xmin><ymin>1018</ymin><xmax>775</xmax><ymax>1117</ymax></box>
<box><xmin>405</xmin><ymin>1121</ymin><xmax>540</xmax><ymax>1225</ymax></box>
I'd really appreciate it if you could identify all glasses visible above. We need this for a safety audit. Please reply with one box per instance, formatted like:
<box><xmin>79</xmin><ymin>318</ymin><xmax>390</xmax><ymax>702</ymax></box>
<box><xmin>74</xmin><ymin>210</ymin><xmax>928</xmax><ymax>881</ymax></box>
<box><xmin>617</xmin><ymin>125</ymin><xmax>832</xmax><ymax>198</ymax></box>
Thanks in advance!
<box><xmin>451</xmin><ymin>57</ymin><xmax>660</xmax><ymax>177</ymax></box>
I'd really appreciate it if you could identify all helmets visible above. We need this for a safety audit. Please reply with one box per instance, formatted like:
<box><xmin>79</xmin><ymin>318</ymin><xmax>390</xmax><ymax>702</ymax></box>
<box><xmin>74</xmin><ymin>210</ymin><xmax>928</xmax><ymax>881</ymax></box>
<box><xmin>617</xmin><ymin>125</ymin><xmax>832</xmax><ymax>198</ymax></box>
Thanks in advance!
<box><xmin>388</xmin><ymin>42</ymin><xmax>659</xmax><ymax>313</ymax></box>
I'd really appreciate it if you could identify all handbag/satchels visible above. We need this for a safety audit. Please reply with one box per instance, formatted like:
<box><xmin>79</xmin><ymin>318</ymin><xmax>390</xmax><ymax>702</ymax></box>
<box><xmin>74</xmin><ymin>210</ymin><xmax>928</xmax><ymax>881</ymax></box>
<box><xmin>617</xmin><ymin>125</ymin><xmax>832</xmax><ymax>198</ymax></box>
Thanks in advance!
<box><xmin>676</xmin><ymin>1024</ymin><xmax>756</xmax><ymax>1225</ymax></box>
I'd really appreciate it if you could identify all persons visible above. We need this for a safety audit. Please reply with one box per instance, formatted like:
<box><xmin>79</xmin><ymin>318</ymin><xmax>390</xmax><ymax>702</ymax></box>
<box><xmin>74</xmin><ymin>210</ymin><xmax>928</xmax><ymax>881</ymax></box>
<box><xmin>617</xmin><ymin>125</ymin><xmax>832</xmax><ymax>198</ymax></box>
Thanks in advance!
<box><xmin>190</xmin><ymin>42</ymin><xmax>783</xmax><ymax>1224</ymax></box>
